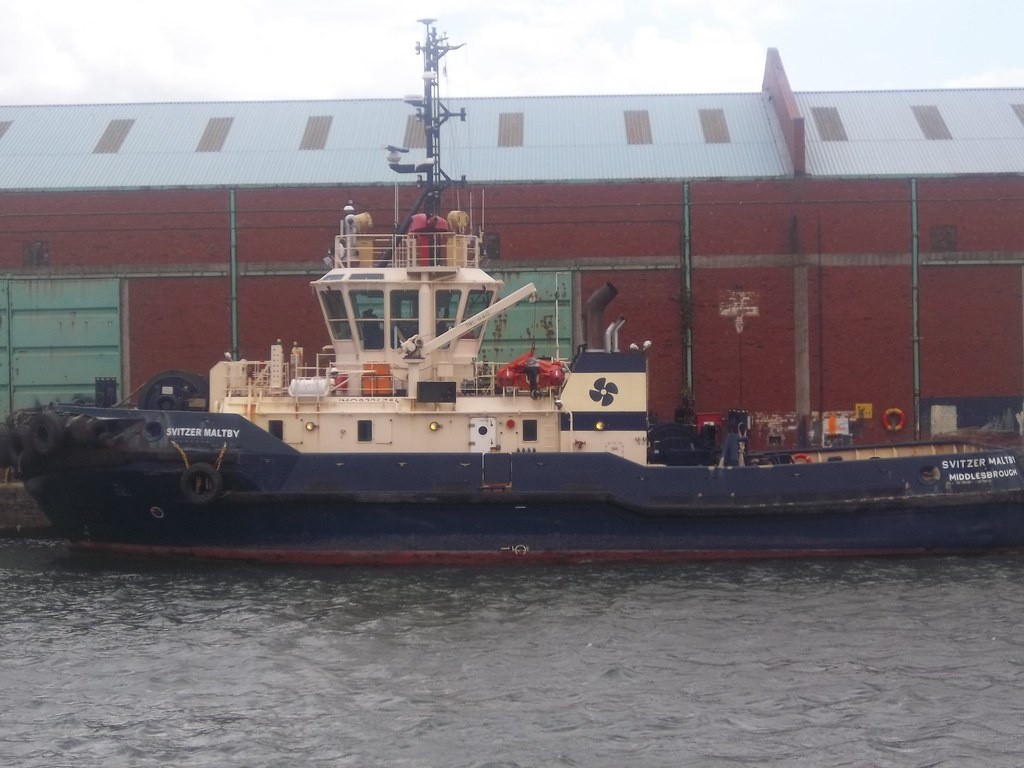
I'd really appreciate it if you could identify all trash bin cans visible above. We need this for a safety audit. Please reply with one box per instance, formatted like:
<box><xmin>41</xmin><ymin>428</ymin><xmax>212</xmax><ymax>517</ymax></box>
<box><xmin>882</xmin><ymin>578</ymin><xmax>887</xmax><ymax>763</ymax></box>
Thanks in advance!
<box><xmin>94</xmin><ymin>376</ymin><xmax>117</xmax><ymax>408</ymax></box>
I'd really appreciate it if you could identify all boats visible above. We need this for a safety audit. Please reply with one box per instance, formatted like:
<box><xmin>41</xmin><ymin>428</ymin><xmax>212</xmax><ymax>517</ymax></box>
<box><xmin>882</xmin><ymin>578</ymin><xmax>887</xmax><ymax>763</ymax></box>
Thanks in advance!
<box><xmin>0</xmin><ymin>15</ymin><xmax>1024</xmax><ymax>565</ymax></box>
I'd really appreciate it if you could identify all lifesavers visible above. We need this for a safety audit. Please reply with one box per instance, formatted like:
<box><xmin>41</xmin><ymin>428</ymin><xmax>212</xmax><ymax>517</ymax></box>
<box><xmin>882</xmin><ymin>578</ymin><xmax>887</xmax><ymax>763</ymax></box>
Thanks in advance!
<box><xmin>180</xmin><ymin>462</ymin><xmax>223</xmax><ymax>506</ymax></box>
<box><xmin>0</xmin><ymin>410</ymin><xmax>67</xmax><ymax>473</ymax></box>
<box><xmin>882</xmin><ymin>408</ymin><xmax>905</xmax><ymax>432</ymax></box>
<box><xmin>790</xmin><ymin>453</ymin><xmax>811</xmax><ymax>464</ymax></box>
